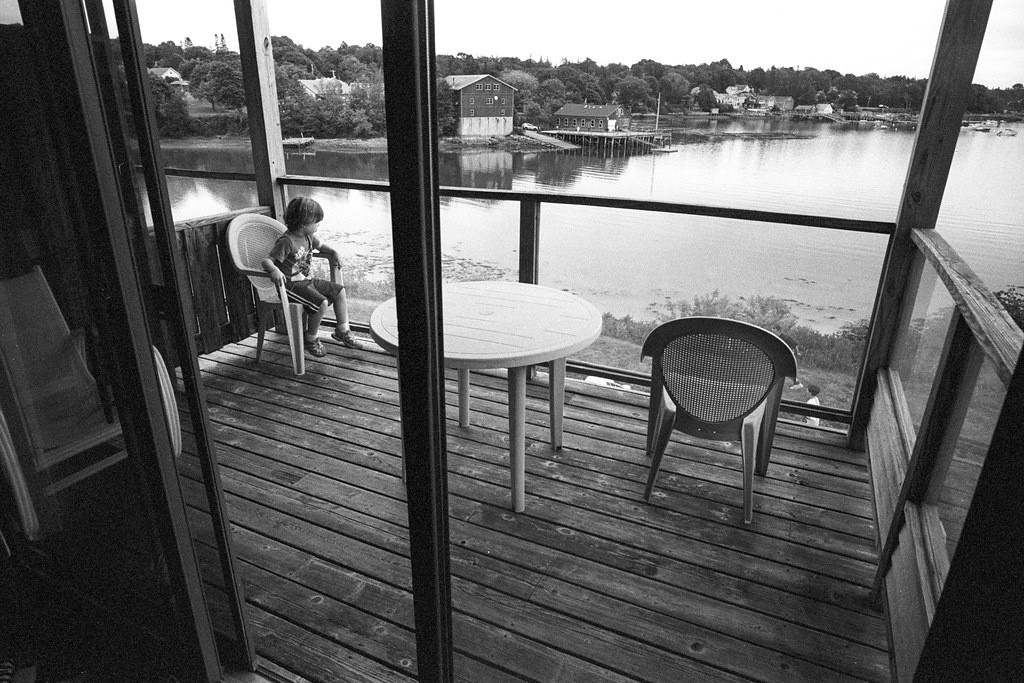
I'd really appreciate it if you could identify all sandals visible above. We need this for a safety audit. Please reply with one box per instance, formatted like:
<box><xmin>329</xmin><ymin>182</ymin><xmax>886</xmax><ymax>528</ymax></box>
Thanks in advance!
<box><xmin>331</xmin><ymin>326</ymin><xmax>364</xmax><ymax>349</ymax></box>
<box><xmin>303</xmin><ymin>330</ymin><xmax>327</xmax><ymax>357</ymax></box>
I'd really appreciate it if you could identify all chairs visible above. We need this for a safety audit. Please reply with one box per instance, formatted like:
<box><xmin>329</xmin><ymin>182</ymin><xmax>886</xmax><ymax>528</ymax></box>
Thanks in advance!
<box><xmin>639</xmin><ymin>315</ymin><xmax>799</xmax><ymax>526</ymax></box>
<box><xmin>0</xmin><ymin>266</ymin><xmax>184</xmax><ymax>499</ymax></box>
<box><xmin>226</xmin><ymin>213</ymin><xmax>344</xmax><ymax>376</ymax></box>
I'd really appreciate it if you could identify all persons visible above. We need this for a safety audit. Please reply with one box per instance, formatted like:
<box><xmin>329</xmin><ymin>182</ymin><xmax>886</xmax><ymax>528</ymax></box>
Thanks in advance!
<box><xmin>772</xmin><ymin>325</ymin><xmax>803</xmax><ymax>390</ymax></box>
<box><xmin>262</xmin><ymin>197</ymin><xmax>363</xmax><ymax>358</ymax></box>
<box><xmin>805</xmin><ymin>385</ymin><xmax>821</xmax><ymax>426</ymax></box>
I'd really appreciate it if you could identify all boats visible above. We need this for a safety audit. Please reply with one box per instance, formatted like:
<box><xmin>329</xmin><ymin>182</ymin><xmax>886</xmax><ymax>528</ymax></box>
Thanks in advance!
<box><xmin>975</xmin><ymin>126</ymin><xmax>992</xmax><ymax>132</ymax></box>
<box><xmin>996</xmin><ymin>128</ymin><xmax>1018</xmax><ymax>137</ymax></box>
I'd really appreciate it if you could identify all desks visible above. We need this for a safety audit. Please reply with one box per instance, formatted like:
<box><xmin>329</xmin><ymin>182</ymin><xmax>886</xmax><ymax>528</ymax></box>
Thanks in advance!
<box><xmin>368</xmin><ymin>279</ymin><xmax>605</xmax><ymax>513</ymax></box>
